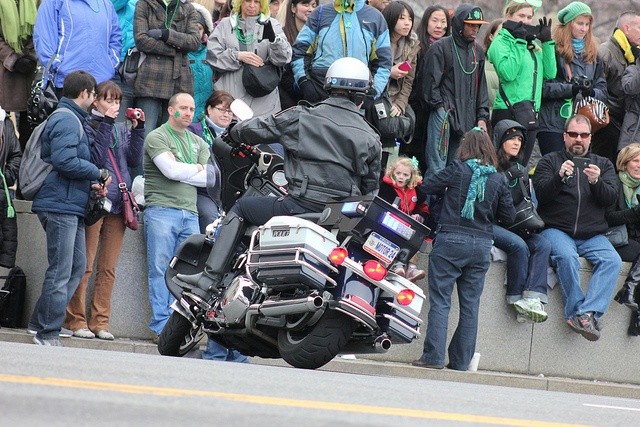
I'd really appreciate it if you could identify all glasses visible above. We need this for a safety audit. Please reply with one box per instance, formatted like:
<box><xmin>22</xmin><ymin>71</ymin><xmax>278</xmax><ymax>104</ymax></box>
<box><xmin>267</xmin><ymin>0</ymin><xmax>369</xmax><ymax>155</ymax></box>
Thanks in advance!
<box><xmin>566</xmin><ymin>132</ymin><xmax>591</xmax><ymax>137</ymax></box>
<box><xmin>213</xmin><ymin>106</ymin><xmax>234</xmax><ymax>115</ymax></box>
<box><xmin>505</xmin><ymin>0</ymin><xmax>542</xmax><ymax>13</ymax></box>
<box><xmin>88</xmin><ymin>91</ymin><xmax>97</xmax><ymax>99</ymax></box>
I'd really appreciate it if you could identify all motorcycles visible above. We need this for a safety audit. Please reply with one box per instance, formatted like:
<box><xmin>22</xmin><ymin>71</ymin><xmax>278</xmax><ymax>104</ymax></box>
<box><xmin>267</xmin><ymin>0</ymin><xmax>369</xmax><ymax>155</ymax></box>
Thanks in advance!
<box><xmin>157</xmin><ymin>99</ymin><xmax>434</xmax><ymax>369</ymax></box>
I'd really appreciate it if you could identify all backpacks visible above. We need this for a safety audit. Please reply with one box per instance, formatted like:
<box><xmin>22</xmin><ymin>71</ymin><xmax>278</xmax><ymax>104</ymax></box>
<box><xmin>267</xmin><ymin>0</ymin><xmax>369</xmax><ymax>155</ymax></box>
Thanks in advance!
<box><xmin>18</xmin><ymin>107</ymin><xmax>83</xmax><ymax>200</ymax></box>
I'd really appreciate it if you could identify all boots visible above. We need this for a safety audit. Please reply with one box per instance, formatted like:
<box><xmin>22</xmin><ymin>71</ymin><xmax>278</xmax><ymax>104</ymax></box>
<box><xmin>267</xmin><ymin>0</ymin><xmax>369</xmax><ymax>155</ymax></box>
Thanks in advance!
<box><xmin>628</xmin><ymin>286</ymin><xmax>640</xmax><ymax>336</ymax></box>
<box><xmin>170</xmin><ymin>211</ymin><xmax>247</xmax><ymax>303</ymax></box>
<box><xmin>614</xmin><ymin>254</ymin><xmax>638</xmax><ymax>312</ymax></box>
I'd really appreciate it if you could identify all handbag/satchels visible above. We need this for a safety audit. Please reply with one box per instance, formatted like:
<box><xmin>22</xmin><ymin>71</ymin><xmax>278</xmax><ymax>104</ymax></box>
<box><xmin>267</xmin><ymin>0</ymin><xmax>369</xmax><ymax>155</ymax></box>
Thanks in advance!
<box><xmin>1</xmin><ymin>266</ymin><xmax>26</xmax><ymax>327</ymax></box>
<box><xmin>574</xmin><ymin>96</ymin><xmax>610</xmax><ymax>135</ymax></box>
<box><xmin>506</xmin><ymin>199</ymin><xmax>545</xmax><ymax>232</ymax></box>
<box><xmin>281</xmin><ymin>55</ymin><xmax>311</xmax><ymax>98</ymax></box>
<box><xmin>606</xmin><ymin>224</ymin><xmax>630</xmax><ymax>249</ymax></box>
<box><xmin>242</xmin><ymin>61</ymin><xmax>280</xmax><ymax>97</ymax></box>
<box><xmin>108</xmin><ymin>146</ymin><xmax>139</xmax><ymax>230</ymax></box>
<box><xmin>27</xmin><ymin>82</ymin><xmax>58</xmax><ymax>129</ymax></box>
<box><xmin>512</xmin><ymin>100</ymin><xmax>540</xmax><ymax>130</ymax></box>
<box><xmin>372</xmin><ymin>97</ymin><xmax>416</xmax><ymax>138</ymax></box>
<box><xmin>117</xmin><ymin>45</ymin><xmax>147</xmax><ymax>87</ymax></box>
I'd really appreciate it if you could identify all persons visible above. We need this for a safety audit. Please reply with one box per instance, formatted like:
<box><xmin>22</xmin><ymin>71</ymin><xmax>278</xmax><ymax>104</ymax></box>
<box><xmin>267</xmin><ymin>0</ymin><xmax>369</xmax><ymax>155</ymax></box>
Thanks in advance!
<box><xmin>535</xmin><ymin>1</ymin><xmax>608</xmax><ymax>157</ymax></box>
<box><xmin>381</xmin><ymin>1</ymin><xmax>420</xmax><ymax>175</ymax></box>
<box><xmin>130</xmin><ymin>0</ymin><xmax>200</xmax><ymax>135</ymax></box>
<box><xmin>185</xmin><ymin>90</ymin><xmax>237</xmax><ymax>234</ymax></box>
<box><xmin>31</xmin><ymin>0</ymin><xmax>122</xmax><ymax>115</ymax></box>
<box><xmin>616</xmin><ymin>56</ymin><xmax>639</xmax><ymax>155</ymax></box>
<box><xmin>420</xmin><ymin>1</ymin><xmax>490</xmax><ymax>168</ymax></box>
<box><xmin>290</xmin><ymin>0</ymin><xmax>394</xmax><ymax>114</ymax></box>
<box><xmin>484</xmin><ymin>0</ymin><xmax>558</xmax><ymax>167</ymax></box>
<box><xmin>213</xmin><ymin>0</ymin><xmax>233</xmax><ymax>31</ymax></box>
<box><xmin>269</xmin><ymin>0</ymin><xmax>284</xmax><ymax>18</ymax></box>
<box><xmin>111</xmin><ymin>0</ymin><xmax>138</xmax><ymax>122</ymax></box>
<box><xmin>379</xmin><ymin>156</ymin><xmax>430</xmax><ymax>227</ymax></box>
<box><xmin>0</xmin><ymin>0</ymin><xmax>42</xmax><ymax>151</ymax></box>
<box><xmin>591</xmin><ymin>10</ymin><xmax>640</xmax><ymax>158</ymax></box>
<box><xmin>398</xmin><ymin>3</ymin><xmax>452</xmax><ymax>176</ymax></box>
<box><xmin>366</xmin><ymin>1</ymin><xmax>391</xmax><ymax>13</ymax></box>
<box><xmin>61</xmin><ymin>79</ymin><xmax>146</xmax><ymax>341</ymax></box>
<box><xmin>491</xmin><ymin>118</ymin><xmax>552</xmax><ymax>325</ymax></box>
<box><xmin>187</xmin><ymin>3</ymin><xmax>214</xmax><ymax>123</ymax></box>
<box><xmin>204</xmin><ymin>0</ymin><xmax>292</xmax><ymax>119</ymax></box>
<box><xmin>610</xmin><ymin>142</ymin><xmax>640</xmax><ymax>336</ymax></box>
<box><xmin>483</xmin><ymin>18</ymin><xmax>502</xmax><ymax>120</ymax></box>
<box><xmin>26</xmin><ymin>69</ymin><xmax>112</xmax><ymax>347</ymax></box>
<box><xmin>1</xmin><ymin>105</ymin><xmax>23</xmax><ymax>189</ymax></box>
<box><xmin>142</xmin><ymin>92</ymin><xmax>218</xmax><ymax>343</ymax></box>
<box><xmin>170</xmin><ymin>55</ymin><xmax>384</xmax><ymax>303</ymax></box>
<box><xmin>212</xmin><ymin>0</ymin><xmax>225</xmax><ymax>23</ymax></box>
<box><xmin>533</xmin><ymin>114</ymin><xmax>620</xmax><ymax>342</ymax></box>
<box><xmin>276</xmin><ymin>0</ymin><xmax>319</xmax><ymax>111</ymax></box>
<box><xmin>412</xmin><ymin>128</ymin><xmax>517</xmax><ymax>370</ymax></box>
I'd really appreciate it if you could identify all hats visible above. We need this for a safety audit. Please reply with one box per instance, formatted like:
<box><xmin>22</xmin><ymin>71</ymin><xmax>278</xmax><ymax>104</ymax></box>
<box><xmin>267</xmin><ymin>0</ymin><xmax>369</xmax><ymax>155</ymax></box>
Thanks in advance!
<box><xmin>501</xmin><ymin>128</ymin><xmax>524</xmax><ymax>145</ymax></box>
<box><xmin>557</xmin><ymin>1</ymin><xmax>591</xmax><ymax>26</ymax></box>
<box><xmin>464</xmin><ymin>8</ymin><xmax>488</xmax><ymax>24</ymax></box>
<box><xmin>196</xmin><ymin>12</ymin><xmax>206</xmax><ymax>30</ymax></box>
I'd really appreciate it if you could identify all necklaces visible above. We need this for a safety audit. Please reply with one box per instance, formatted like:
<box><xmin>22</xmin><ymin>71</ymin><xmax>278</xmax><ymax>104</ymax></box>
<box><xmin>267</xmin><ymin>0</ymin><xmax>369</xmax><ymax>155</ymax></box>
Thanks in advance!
<box><xmin>452</xmin><ymin>36</ymin><xmax>477</xmax><ymax>75</ymax></box>
<box><xmin>164</xmin><ymin>0</ymin><xmax>179</xmax><ymax>30</ymax></box>
<box><xmin>108</xmin><ymin>123</ymin><xmax>119</xmax><ymax>148</ymax></box>
<box><xmin>507</xmin><ymin>163</ymin><xmax>519</xmax><ymax>187</ymax></box>
<box><xmin>165</xmin><ymin>120</ymin><xmax>193</xmax><ymax>164</ymax></box>
<box><xmin>235</xmin><ymin>13</ymin><xmax>262</xmax><ymax>45</ymax></box>
<box><xmin>342</xmin><ymin>1</ymin><xmax>357</xmax><ymax>28</ymax></box>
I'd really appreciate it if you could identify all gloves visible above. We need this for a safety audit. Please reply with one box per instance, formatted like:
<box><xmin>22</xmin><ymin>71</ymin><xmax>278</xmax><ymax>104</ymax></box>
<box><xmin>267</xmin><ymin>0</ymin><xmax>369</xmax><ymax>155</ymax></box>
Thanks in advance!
<box><xmin>536</xmin><ymin>16</ymin><xmax>552</xmax><ymax>43</ymax></box>
<box><xmin>257</xmin><ymin>19</ymin><xmax>275</xmax><ymax>42</ymax></box>
<box><xmin>633</xmin><ymin>193</ymin><xmax>640</xmax><ymax>222</ymax></box>
<box><xmin>15</xmin><ymin>55</ymin><xmax>36</xmax><ymax>73</ymax></box>
<box><xmin>504</xmin><ymin>167</ymin><xmax>524</xmax><ymax>182</ymax></box>
<box><xmin>514</xmin><ymin>22</ymin><xmax>528</xmax><ymax>44</ymax></box>
<box><xmin>149</xmin><ymin>29</ymin><xmax>162</xmax><ymax>40</ymax></box>
<box><xmin>220</xmin><ymin>123</ymin><xmax>238</xmax><ymax>148</ymax></box>
<box><xmin>572</xmin><ymin>84</ymin><xmax>595</xmax><ymax>98</ymax></box>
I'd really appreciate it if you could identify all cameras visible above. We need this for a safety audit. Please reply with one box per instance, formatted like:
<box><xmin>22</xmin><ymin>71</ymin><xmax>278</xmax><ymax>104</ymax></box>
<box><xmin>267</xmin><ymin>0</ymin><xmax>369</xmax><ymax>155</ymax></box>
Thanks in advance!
<box><xmin>85</xmin><ymin>196</ymin><xmax>113</xmax><ymax>226</ymax></box>
<box><xmin>124</xmin><ymin>107</ymin><xmax>142</xmax><ymax>120</ymax></box>
<box><xmin>374</xmin><ymin>103</ymin><xmax>388</xmax><ymax>120</ymax></box>
<box><xmin>571</xmin><ymin>75</ymin><xmax>592</xmax><ymax>88</ymax></box>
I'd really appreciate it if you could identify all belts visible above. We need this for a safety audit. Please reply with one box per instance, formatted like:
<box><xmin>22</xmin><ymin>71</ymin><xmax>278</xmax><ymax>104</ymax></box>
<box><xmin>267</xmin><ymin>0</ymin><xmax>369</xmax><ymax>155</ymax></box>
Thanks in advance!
<box><xmin>288</xmin><ymin>181</ymin><xmax>350</xmax><ymax>204</ymax></box>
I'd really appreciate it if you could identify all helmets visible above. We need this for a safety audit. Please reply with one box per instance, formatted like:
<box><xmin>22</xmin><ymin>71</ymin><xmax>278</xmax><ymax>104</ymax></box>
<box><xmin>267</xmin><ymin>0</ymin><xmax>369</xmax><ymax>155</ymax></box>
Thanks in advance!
<box><xmin>323</xmin><ymin>57</ymin><xmax>377</xmax><ymax>92</ymax></box>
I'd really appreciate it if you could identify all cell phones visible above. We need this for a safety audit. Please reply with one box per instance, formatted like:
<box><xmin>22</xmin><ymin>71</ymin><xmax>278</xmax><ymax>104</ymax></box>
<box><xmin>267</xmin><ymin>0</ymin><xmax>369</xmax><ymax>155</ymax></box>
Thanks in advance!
<box><xmin>572</xmin><ymin>157</ymin><xmax>591</xmax><ymax>168</ymax></box>
<box><xmin>397</xmin><ymin>62</ymin><xmax>412</xmax><ymax>72</ymax></box>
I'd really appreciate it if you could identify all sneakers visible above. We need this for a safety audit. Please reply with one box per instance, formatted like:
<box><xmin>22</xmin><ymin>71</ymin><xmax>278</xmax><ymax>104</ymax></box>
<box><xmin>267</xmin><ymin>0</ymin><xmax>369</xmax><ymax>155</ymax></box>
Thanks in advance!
<box><xmin>567</xmin><ymin>313</ymin><xmax>600</xmax><ymax>341</ymax></box>
<box><xmin>513</xmin><ymin>297</ymin><xmax>548</xmax><ymax>323</ymax></box>
<box><xmin>406</xmin><ymin>268</ymin><xmax>425</xmax><ymax>283</ymax></box>
<box><xmin>594</xmin><ymin>318</ymin><xmax>603</xmax><ymax>330</ymax></box>
<box><xmin>412</xmin><ymin>358</ymin><xmax>443</xmax><ymax>369</ymax></box>
<box><xmin>27</xmin><ymin>321</ymin><xmax>73</xmax><ymax>337</ymax></box>
<box><xmin>395</xmin><ymin>268</ymin><xmax>405</xmax><ymax>277</ymax></box>
<box><xmin>72</xmin><ymin>328</ymin><xmax>95</xmax><ymax>339</ymax></box>
<box><xmin>516</xmin><ymin>312</ymin><xmax>528</xmax><ymax>323</ymax></box>
<box><xmin>446</xmin><ymin>363</ymin><xmax>453</xmax><ymax>369</ymax></box>
<box><xmin>32</xmin><ymin>335</ymin><xmax>65</xmax><ymax>346</ymax></box>
<box><xmin>96</xmin><ymin>329</ymin><xmax>115</xmax><ymax>340</ymax></box>
